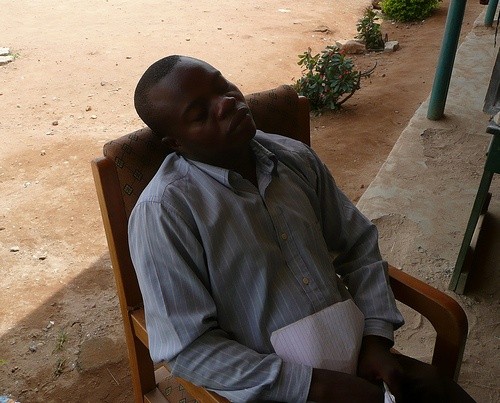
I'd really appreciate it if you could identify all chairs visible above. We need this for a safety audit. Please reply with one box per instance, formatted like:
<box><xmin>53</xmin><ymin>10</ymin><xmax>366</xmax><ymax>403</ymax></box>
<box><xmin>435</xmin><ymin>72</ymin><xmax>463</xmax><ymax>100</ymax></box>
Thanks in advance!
<box><xmin>90</xmin><ymin>85</ymin><xmax>469</xmax><ymax>403</ymax></box>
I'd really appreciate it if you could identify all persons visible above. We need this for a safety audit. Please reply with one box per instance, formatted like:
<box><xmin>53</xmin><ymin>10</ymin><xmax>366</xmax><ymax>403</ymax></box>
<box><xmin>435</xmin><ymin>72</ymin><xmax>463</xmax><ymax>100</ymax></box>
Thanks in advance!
<box><xmin>127</xmin><ymin>55</ymin><xmax>477</xmax><ymax>403</ymax></box>
